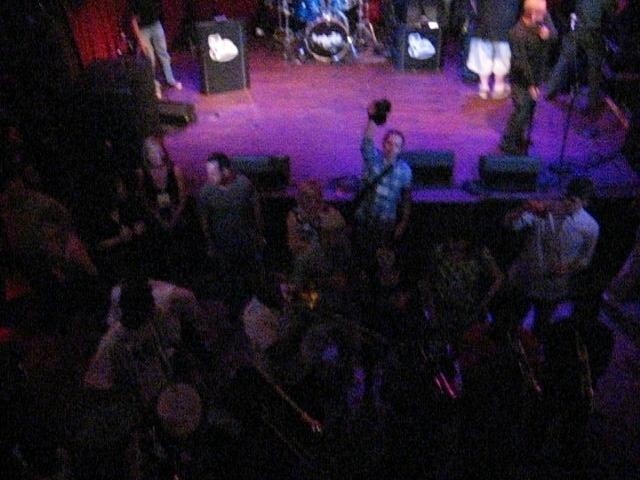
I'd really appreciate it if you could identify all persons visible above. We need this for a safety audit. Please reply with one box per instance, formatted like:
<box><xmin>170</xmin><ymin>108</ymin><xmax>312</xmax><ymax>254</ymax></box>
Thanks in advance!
<box><xmin>542</xmin><ymin>1</ymin><xmax>627</xmax><ymax>117</ymax></box>
<box><xmin>2</xmin><ymin>101</ymin><xmax>599</xmax><ymax>478</ymax></box>
<box><xmin>498</xmin><ymin>0</ymin><xmax>558</xmax><ymax>156</ymax></box>
<box><xmin>465</xmin><ymin>1</ymin><xmax>523</xmax><ymax>101</ymax></box>
<box><xmin>127</xmin><ymin>1</ymin><xmax>184</xmax><ymax>99</ymax></box>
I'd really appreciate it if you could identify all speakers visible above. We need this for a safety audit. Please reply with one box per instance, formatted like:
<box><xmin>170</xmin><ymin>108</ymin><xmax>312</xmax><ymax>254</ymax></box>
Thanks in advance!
<box><xmin>199</xmin><ymin>20</ymin><xmax>248</xmax><ymax>95</ymax></box>
<box><xmin>397</xmin><ymin>24</ymin><xmax>445</xmax><ymax>72</ymax></box>
<box><xmin>406</xmin><ymin>148</ymin><xmax>453</xmax><ymax>188</ymax></box>
<box><xmin>233</xmin><ymin>147</ymin><xmax>292</xmax><ymax>190</ymax></box>
<box><xmin>483</xmin><ymin>151</ymin><xmax>542</xmax><ymax>192</ymax></box>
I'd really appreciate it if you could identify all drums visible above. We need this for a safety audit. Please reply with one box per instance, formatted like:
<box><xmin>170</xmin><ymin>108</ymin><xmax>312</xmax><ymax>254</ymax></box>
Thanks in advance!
<box><xmin>304</xmin><ymin>10</ymin><xmax>350</xmax><ymax>63</ymax></box>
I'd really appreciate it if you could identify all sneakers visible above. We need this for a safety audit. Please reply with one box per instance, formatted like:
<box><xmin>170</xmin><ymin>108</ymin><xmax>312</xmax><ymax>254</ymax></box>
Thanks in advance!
<box><xmin>476</xmin><ymin>83</ymin><xmax>511</xmax><ymax>103</ymax></box>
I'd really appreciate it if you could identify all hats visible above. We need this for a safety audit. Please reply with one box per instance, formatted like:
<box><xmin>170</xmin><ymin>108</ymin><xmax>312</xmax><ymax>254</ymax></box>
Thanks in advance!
<box><xmin>563</xmin><ymin>178</ymin><xmax>593</xmax><ymax>200</ymax></box>
<box><xmin>370</xmin><ymin>98</ymin><xmax>391</xmax><ymax>126</ymax></box>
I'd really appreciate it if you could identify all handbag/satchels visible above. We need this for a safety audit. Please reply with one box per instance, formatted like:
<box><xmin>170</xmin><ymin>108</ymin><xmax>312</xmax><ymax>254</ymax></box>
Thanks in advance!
<box><xmin>339</xmin><ymin>181</ymin><xmax>373</xmax><ymax>224</ymax></box>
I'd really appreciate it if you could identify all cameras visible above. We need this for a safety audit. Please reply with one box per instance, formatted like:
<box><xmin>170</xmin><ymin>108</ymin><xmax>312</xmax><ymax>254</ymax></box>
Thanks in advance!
<box><xmin>372</xmin><ymin>100</ymin><xmax>392</xmax><ymax>123</ymax></box>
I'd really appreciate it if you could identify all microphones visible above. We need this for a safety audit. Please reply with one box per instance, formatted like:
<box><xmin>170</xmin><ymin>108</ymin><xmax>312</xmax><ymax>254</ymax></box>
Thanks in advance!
<box><xmin>567</xmin><ymin>13</ymin><xmax>579</xmax><ymax>34</ymax></box>
